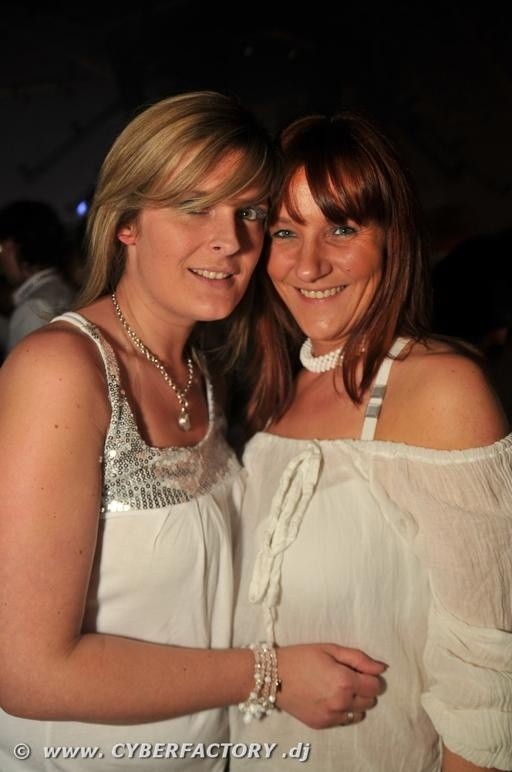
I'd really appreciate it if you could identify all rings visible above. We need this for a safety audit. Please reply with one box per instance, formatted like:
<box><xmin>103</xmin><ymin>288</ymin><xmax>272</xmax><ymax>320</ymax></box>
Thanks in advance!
<box><xmin>343</xmin><ymin>711</ymin><xmax>355</xmax><ymax>726</ymax></box>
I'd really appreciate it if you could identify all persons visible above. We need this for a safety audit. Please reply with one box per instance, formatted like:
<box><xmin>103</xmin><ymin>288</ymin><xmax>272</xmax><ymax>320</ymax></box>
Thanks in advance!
<box><xmin>234</xmin><ymin>113</ymin><xmax>511</xmax><ymax>772</ymax></box>
<box><xmin>0</xmin><ymin>90</ymin><xmax>387</xmax><ymax>772</ymax></box>
<box><xmin>0</xmin><ymin>197</ymin><xmax>76</xmax><ymax>363</ymax></box>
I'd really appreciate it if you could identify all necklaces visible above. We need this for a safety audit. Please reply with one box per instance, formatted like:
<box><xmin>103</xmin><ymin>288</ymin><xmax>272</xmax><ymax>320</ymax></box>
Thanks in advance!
<box><xmin>111</xmin><ymin>286</ymin><xmax>196</xmax><ymax>431</ymax></box>
<box><xmin>297</xmin><ymin>337</ymin><xmax>370</xmax><ymax>375</ymax></box>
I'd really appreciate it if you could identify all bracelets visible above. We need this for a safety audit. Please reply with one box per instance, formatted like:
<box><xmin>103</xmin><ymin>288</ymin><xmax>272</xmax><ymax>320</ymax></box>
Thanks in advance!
<box><xmin>239</xmin><ymin>635</ymin><xmax>281</xmax><ymax>725</ymax></box>
